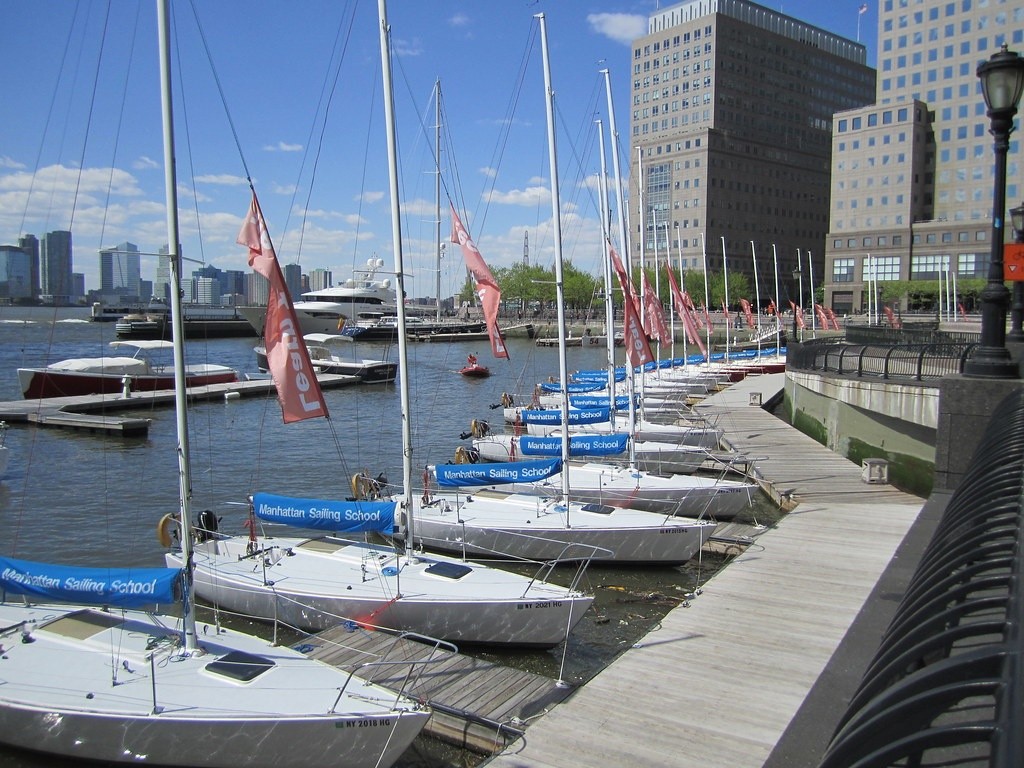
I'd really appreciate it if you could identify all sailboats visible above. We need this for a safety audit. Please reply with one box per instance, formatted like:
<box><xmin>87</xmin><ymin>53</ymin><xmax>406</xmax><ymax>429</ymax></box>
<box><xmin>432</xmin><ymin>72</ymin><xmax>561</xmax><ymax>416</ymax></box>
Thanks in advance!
<box><xmin>0</xmin><ymin>1</ymin><xmax>953</xmax><ymax>768</ymax></box>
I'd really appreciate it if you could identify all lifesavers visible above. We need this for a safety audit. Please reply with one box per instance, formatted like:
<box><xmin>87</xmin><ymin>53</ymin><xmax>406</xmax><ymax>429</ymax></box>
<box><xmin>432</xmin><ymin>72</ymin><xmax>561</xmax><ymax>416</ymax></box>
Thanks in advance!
<box><xmin>501</xmin><ymin>391</ymin><xmax>510</xmax><ymax>409</ymax></box>
<box><xmin>469</xmin><ymin>417</ymin><xmax>483</xmax><ymax>440</ymax></box>
<box><xmin>350</xmin><ymin>470</ymin><xmax>372</xmax><ymax>500</ymax></box>
<box><xmin>453</xmin><ymin>445</ymin><xmax>468</xmax><ymax>466</ymax></box>
<box><xmin>156</xmin><ymin>508</ymin><xmax>183</xmax><ymax>553</ymax></box>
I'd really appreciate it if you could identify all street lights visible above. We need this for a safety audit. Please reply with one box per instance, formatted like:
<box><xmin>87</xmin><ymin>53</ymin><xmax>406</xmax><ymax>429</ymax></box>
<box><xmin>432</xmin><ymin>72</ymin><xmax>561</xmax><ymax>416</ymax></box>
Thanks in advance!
<box><xmin>962</xmin><ymin>41</ymin><xmax>1023</xmax><ymax>379</ymax></box>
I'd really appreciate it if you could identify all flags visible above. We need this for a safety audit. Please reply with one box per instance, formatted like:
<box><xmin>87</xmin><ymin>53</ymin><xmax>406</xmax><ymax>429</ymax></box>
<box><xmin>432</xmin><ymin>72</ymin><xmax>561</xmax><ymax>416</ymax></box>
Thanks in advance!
<box><xmin>859</xmin><ymin>4</ymin><xmax>867</xmax><ymax>13</ymax></box>
